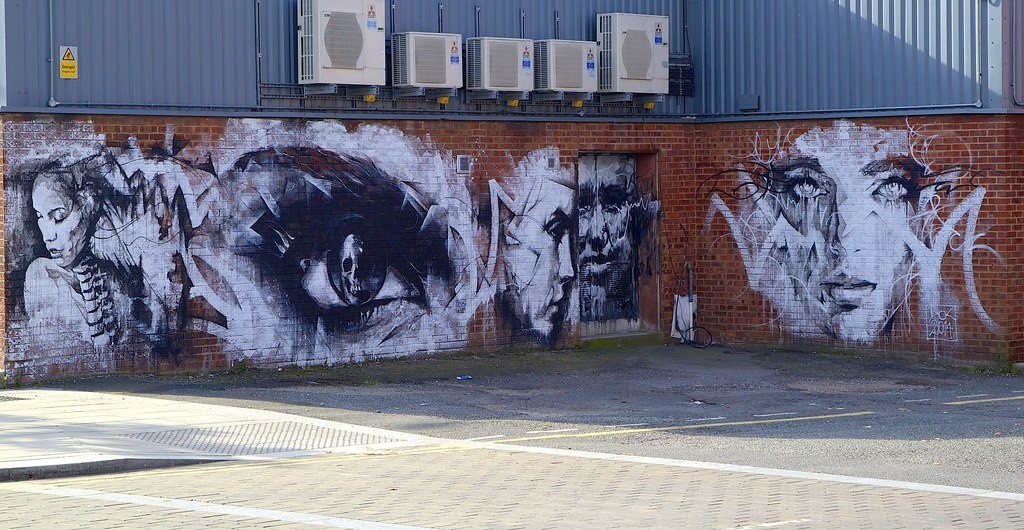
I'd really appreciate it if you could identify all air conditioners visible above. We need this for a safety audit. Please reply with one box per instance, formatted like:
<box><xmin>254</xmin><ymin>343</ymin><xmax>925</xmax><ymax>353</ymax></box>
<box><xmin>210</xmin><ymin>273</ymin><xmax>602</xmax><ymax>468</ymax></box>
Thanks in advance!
<box><xmin>297</xmin><ymin>0</ymin><xmax>386</xmax><ymax>86</ymax></box>
<box><xmin>466</xmin><ymin>37</ymin><xmax>535</xmax><ymax>93</ymax></box>
<box><xmin>596</xmin><ymin>12</ymin><xmax>670</xmax><ymax>96</ymax></box>
<box><xmin>534</xmin><ymin>40</ymin><xmax>599</xmax><ymax>93</ymax></box>
<box><xmin>390</xmin><ymin>31</ymin><xmax>463</xmax><ymax>88</ymax></box>
